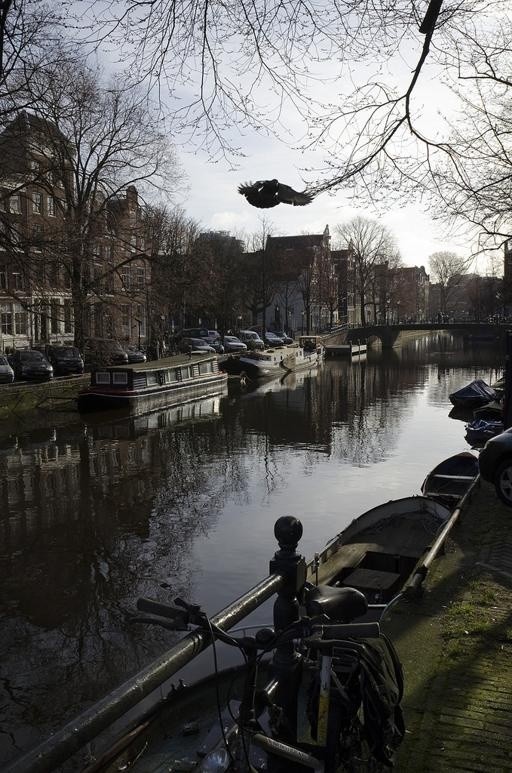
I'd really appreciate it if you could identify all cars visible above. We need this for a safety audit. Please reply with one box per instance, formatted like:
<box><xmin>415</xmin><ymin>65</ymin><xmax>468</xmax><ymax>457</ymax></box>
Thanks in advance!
<box><xmin>480</xmin><ymin>423</ymin><xmax>511</xmax><ymax>506</ymax></box>
<box><xmin>1</xmin><ymin>321</ymin><xmax>295</xmax><ymax>382</ymax></box>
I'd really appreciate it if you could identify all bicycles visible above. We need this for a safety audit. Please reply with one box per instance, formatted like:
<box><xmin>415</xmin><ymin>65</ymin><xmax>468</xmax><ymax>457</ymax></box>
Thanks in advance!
<box><xmin>135</xmin><ymin>580</ymin><xmax>407</xmax><ymax>772</ymax></box>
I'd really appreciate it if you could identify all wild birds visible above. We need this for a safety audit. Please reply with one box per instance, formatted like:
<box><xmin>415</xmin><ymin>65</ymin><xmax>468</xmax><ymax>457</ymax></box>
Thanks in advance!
<box><xmin>237</xmin><ymin>178</ymin><xmax>314</xmax><ymax>209</ymax></box>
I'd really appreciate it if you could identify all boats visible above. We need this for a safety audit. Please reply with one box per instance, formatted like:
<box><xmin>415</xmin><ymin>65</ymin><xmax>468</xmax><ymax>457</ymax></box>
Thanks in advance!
<box><xmin>237</xmin><ymin>365</ymin><xmax>321</xmax><ymax>396</ymax></box>
<box><xmin>81</xmin><ymin>394</ymin><xmax>220</xmax><ymax>441</ymax></box>
<box><xmin>83</xmin><ymin>349</ymin><xmax>229</xmax><ymax>411</ymax></box>
<box><xmin>73</xmin><ymin>661</ymin><xmax>277</xmax><ymax>772</ymax></box>
<box><xmin>237</xmin><ymin>334</ymin><xmax>326</xmax><ymax>380</ymax></box>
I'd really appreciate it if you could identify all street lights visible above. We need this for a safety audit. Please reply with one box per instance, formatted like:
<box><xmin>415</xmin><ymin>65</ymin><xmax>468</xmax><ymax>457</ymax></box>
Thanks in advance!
<box><xmin>161</xmin><ymin>312</ymin><xmax>169</xmax><ymax>341</ymax></box>
<box><xmin>385</xmin><ymin>298</ymin><xmax>391</xmax><ymax>324</ymax></box>
<box><xmin>237</xmin><ymin>314</ymin><xmax>242</xmax><ymax>334</ymax></box>
<box><xmin>395</xmin><ymin>298</ymin><xmax>400</xmax><ymax>324</ymax></box>
<box><xmin>300</xmin><ymin>310</ymin><xmax>305</xmax><ymax>335</ymax></box>
